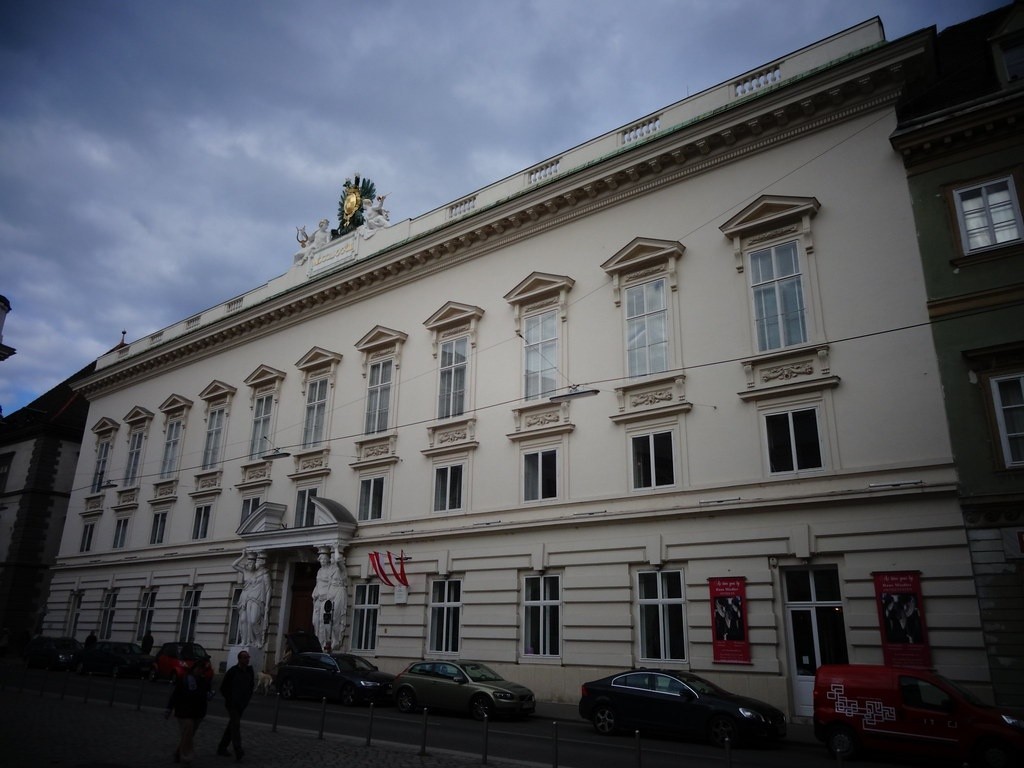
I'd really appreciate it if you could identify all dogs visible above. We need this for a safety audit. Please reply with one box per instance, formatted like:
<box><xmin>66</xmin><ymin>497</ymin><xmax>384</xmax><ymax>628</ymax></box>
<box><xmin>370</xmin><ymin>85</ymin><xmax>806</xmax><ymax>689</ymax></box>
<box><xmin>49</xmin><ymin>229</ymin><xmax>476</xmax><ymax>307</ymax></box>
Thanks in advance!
<box><xmin>252</xmin><ymin>670</ymin><xmax>273</xmax><ymax>696</ymax></box>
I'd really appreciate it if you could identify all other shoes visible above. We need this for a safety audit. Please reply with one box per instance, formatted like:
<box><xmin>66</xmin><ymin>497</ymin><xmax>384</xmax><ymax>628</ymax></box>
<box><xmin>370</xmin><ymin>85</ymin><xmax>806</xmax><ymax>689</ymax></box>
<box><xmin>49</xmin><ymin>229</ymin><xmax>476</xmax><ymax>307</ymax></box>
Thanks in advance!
<box><xmin>236</xmin><ymin>750</ymin><xmax>245</xmax><ymax>762</ymax></box>
<box><xmin>217</xmin><ymin>748</ymin><xmax>232</xmax><ymax>756</ymax></box>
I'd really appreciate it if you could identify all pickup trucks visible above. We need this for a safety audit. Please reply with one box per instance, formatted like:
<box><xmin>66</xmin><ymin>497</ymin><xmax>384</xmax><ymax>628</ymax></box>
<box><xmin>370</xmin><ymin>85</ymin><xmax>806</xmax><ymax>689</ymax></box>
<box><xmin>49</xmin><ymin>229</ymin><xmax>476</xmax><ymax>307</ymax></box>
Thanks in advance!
<box><xmin>813</xmin><ymin>664</ymin><xmax>1024</xmax><ymax>768</ymax></box>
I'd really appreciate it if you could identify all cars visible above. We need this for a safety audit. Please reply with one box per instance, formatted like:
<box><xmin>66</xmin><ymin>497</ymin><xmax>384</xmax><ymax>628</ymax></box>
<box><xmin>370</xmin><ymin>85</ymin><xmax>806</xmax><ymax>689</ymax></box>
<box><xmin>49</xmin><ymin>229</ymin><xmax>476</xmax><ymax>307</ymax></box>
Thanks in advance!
<box><xmin>579</xmin><ymin>667</ymin><xmax>786</xmax><ymax>749</ymax></box>
<box><xmin>388</xmin><ymin>659</ymin><xmax>536</xmax><ymax>723</ymax></box>
<box><xmin>91</xmin><ymin>641</ymin><xmax>153</xmax><ymax>679</ymax></box>
<box><xmin>149</xmin><ymin>641</ymin><xmax>213</xmax><ymax>690</ymax></box>
<box><xmin>18</xmin><ymin>633</ymin><xmax>87</xmax><ymax>675</ymax></box>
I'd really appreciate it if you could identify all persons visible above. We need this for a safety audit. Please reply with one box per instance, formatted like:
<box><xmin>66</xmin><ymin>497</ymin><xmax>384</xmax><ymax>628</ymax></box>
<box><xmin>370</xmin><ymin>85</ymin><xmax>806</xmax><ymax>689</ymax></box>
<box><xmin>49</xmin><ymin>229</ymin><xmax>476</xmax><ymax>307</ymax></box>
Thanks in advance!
<box><xmin>292</xmin><ymin>217</ymin><xmax>332</xmax><ymax>266</ymax></box>
<box><xmin>140</xmin><ymin>629</ymin><xmax>154</xmax><ymax>657</ymax></box>
<box><xmin>310</xmin><ymin>553</ymin><xmax>347</xmax><ymax>654</ymax></box>
<box><xmin>167</xmin><ymin>657</ymin><xmax>210</xmax><ymax>766</ymax></box>
<box><xmin>84</xmin><ymin>631</ymin><xmax>98</xmax><ymax>650</ymax></box>
<box><xmin>231</xmin><ymin>548</ymin><xmax>270</xmax><ymax>647</ymax></box>
<box><xmin>358</xmin><ymin>194</ymin><xmax>392</xmax><ymax>238</ymax></box>
<box><xmin>278</xmin><ymin>637</ymin><xmax>299</xmax><ymax>665</ymax></box>
<box><xmin>216</xmin><ymin>650</ymin><xmax>255</xmax><ymax>761</ymax></box>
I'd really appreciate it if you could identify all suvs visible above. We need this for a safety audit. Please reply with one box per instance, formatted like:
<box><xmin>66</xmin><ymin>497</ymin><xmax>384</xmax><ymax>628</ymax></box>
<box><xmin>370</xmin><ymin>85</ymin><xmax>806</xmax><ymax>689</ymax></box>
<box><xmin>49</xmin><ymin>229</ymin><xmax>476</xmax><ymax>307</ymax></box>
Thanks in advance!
<box><xmin>275</xmin><ymin>651</ymin><xmax>398</xmax><ymax>708</ymax></box>
